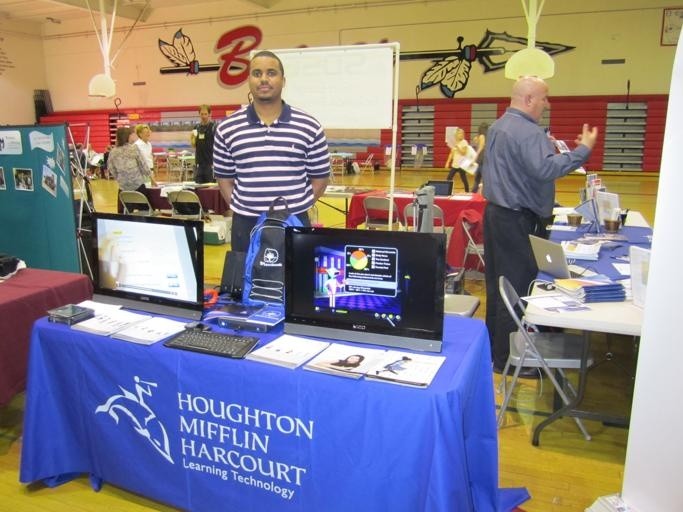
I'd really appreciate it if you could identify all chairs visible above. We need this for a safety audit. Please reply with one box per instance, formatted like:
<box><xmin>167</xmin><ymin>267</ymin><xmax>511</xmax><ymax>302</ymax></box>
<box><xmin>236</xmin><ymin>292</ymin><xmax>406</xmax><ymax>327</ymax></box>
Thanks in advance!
<box><xmin>363</xmin><ymin>197</ymin><xmax>401</xmax><ymax>231</ymax></box>
<box><xmin>496</xmin><ymin>275</ymin><xmax>597</xmax><ymax>440</ymax></box>
<box><xmin>167</xmin><ymin>190</ymin><xmax>211</xmax><ymax>226</ymax></box>
<box><xmin>119</xmin><ymin>190</ymin><xmax>161</xmax><ymax>216</ymax></box>
<box><xmin>166</xmin><ymin>157</ymin><xmax>181</xmax><ymax>180</ymax></box>
<box><xmin>360</xmin><ymin>153</ymin><xmax>374</xmax><ymax>174</ymax></box>
<box><xmin>444</xmin><ymin>292</ymin><xmax>482</xmax><ymax>319</ymax></box>
<box><xmin>330</xmin><ymin>155</ymin><xmax>347</xmax><ymax>177</ymax></box>
<box><xmin>403</xmin><ymin>203</ymin><xmax>445</xmax><ymax>234</ymax></box>
<box><xmin>460</xmin><ymin>210</ymin><xmax>486</xmax><ymax>270</ymax></box>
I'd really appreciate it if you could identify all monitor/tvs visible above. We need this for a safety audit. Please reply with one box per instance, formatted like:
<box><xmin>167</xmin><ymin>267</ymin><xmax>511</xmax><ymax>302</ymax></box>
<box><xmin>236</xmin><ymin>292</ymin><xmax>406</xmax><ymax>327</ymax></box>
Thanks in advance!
<box><xmin>284</xmin><ymin>226</ymin><xmax>446</xmax><ymax>353</ymax></box>
<box><xmin>91</xmin><ymin>212</ymin><xmax>204</xmax><ymax>321</ymax></box>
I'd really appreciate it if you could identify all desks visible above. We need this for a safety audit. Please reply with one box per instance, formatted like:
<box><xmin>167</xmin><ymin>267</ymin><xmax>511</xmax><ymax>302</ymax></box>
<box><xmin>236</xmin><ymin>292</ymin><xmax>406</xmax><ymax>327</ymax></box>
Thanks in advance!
<box><xmin>1</xmin><ymin>268</ymin><xmax>93</xmax><ymax>407</ymax></box>
<box><xmin>328</xmin><ymin>152</ymin><xmax>354</xmax><ymax>175</ymax></box>
<box><xmin>319</xmin><ymin>186</ymin><xmax>369</xmax><ymax>231</ymax></box>
<box><xmin>18</xmin><ymin>296</ymin><xmax>530</xmax><ymax>512</ymax></box>
<box><xmin>182</xmin><ymin>155</ymin><xmax>196</xmax><ymax>180</ymax></box>
<box><xmin>349</xmin><ymin>188</ymin><xmax>488</xmax><ymax>234</ymax></box>
<box><xmin>117</xmin><ymin>182</ymin><xmax>230</xmax><ymax>215</ymax></box>
<box><xmin>525</xmin><ymin>207</ymin><xmax>653</xmax><ymax>445</ymax></box>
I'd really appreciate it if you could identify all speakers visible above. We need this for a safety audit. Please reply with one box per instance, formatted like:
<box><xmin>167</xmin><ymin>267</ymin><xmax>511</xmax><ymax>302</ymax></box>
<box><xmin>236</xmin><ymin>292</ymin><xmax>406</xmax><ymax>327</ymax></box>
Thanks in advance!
<box><xmin>233</xmin><ymin>252</ymin><xmax>247</xmax><ymax>295</ymax></box>
<box><xmin>220</xmin><ymin>250</ymin><xmax>238</xmax><ymax>293</ymax></box>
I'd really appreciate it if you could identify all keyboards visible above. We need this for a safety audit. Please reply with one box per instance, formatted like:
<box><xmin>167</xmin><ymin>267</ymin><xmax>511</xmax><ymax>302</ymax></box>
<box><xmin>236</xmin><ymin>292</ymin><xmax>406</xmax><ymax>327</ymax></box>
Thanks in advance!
<box><xmin>164</xmin><ymin>328</ymin><xmax>260</xmax><ymax>359</ymax></box>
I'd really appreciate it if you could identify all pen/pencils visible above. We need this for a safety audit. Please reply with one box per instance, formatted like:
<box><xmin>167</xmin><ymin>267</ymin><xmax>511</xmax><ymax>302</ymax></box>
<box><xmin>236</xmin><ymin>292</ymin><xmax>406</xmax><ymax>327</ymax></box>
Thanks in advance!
<box><xmin>609</xmin><ymin>257</ymin><xmax>629</xmax><ymax>262</ymax></box>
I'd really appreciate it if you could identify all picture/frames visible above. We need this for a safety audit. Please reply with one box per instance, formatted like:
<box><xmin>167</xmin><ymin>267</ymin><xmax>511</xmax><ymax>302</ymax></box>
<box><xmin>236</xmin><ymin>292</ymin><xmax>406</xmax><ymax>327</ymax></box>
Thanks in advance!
<box><xmin>660</xmin><ymin>8</ymin><xmax>683</xmax><ymax>45</ymax></box>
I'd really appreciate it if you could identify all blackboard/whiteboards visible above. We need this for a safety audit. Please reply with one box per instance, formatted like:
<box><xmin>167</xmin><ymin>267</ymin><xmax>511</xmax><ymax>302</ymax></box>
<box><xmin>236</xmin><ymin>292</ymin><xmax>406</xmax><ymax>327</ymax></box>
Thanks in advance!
<box><xmin>251</xmin><ymin>41</ymin><xmax>400</xmax><ymax>130</ymax></box>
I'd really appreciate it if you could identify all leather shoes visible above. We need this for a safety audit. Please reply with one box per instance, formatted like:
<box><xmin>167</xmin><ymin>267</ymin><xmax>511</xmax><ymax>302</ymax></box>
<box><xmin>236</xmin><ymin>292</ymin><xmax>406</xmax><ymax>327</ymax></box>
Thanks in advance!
<box><xmin>492</xmin><ymin>361</ymin><xmax>546</xmax><ymax>381</ymax></box>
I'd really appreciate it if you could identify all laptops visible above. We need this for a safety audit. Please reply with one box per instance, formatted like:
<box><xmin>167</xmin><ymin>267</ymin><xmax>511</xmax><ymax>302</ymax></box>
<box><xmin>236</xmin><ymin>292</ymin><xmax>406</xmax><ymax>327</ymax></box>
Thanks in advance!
<box><xmin>528</xmin><ymin>234</ymin><xmax>599</xmax><ymax>278</ymax></box>
<box><xmin>428</xmin><ymin>181</ymin><xmax>453</xmax><ymax>199</ymax></box>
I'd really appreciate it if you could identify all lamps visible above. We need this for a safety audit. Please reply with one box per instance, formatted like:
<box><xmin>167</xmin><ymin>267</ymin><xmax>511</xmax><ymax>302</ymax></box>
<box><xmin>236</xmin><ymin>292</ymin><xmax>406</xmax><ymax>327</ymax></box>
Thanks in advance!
<box><xmin>505</xmin><ymin>0</ymin><xmax>555</xmax><ymax>80</ymax></box>
<box><xmin>87</xmin><ymin>0</ymin><xmax>153</xmax><ymax>98</ymax></box>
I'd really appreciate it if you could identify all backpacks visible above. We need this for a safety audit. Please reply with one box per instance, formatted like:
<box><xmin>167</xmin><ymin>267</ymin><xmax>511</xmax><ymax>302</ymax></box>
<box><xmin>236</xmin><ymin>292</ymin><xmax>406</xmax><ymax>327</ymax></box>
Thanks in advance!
<box><xmin>240</xmin><ymin>208</ymin><xmax>303</xmax><ymax>311</ymax></box>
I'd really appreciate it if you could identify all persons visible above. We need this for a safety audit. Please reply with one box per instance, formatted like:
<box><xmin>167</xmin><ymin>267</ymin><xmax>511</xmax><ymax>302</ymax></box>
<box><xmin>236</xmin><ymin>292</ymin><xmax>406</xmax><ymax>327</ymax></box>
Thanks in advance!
<box><xmin>482</xmin><ymin>77</ymin><xmax>598</xmax><ymax>378</ymax></box>
<box><xmin>210</xmin><ymin>49</ymin><xmax>331</xmax><ymax>258</ymax></box>
<box><xmin>443</xmin><ymin>128</ymin><xmax>469</xmax><ymax>192</ymax></box>
<box><xmin>471</xmin><ymin>135</ymin><xmax>486</xmax><ymax>192</ymax></box>
<box><xmin>75</xmin><ymin>122</ymin><xmax>161</xmax><ymax>216</ymax></box>
<box><xmin>192</xmin><ymin>103</ymin><xmax>220</xmax><ymax>184</ymax></box>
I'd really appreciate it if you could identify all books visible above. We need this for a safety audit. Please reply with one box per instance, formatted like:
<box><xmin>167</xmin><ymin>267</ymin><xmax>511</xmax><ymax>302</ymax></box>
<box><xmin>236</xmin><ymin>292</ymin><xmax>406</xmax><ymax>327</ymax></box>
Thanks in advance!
<box><xmin>552</xmin><ymin>272</ymin><xmax>626</xmax><ymax>303</ymax></box>
